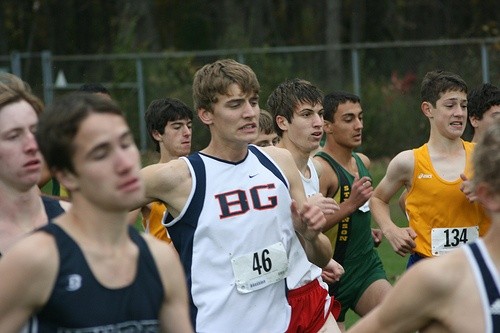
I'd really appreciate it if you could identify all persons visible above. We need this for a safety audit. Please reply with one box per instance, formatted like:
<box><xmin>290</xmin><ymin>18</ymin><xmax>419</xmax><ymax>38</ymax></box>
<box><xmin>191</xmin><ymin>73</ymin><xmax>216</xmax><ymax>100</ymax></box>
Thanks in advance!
<box><xmin>369</xmin><ymin>70</ymin><xmax>483</xmax><ymax>272</ymax></box>
<box><xmin>266</xmin><ymin>78</ymin><xmax>345</xmax><ymax>333</ymax></box>
<box><xmin>347</xmin><ymin>113</ymin><xmax>499</xmax><ymax>333</ymax></box>
<box><xmin>0</xmin><ymin>70</ymin><xmax>73</xmax><ymax>255</ymax></box>
<box><xmin>0</xmin><ymin>90</ymin><xmax>193</xmax><ymax>333</ymax></box>
<box><xmin>127</xmin><ymin>58</ymin><xmax>333</xmax><ymax>333</ymax></box>
<box><xmin>138</xmin><ymin>98</ymin><xmax>192</xmax><ymax>245</ymax></box>
<box><xmin>310</xmin><ymin>89</ymin><xmax>398</xmax><ymax>333</ymax></box>
<box><xmin>247</xmin><ymin>109</ymin><xmax>279</xmax><ymax>148</ymax></box>
<box><xmin>467</xmin><ymin>84</ymin><xmax>499</xmax><ymax>144</ymax></box>
